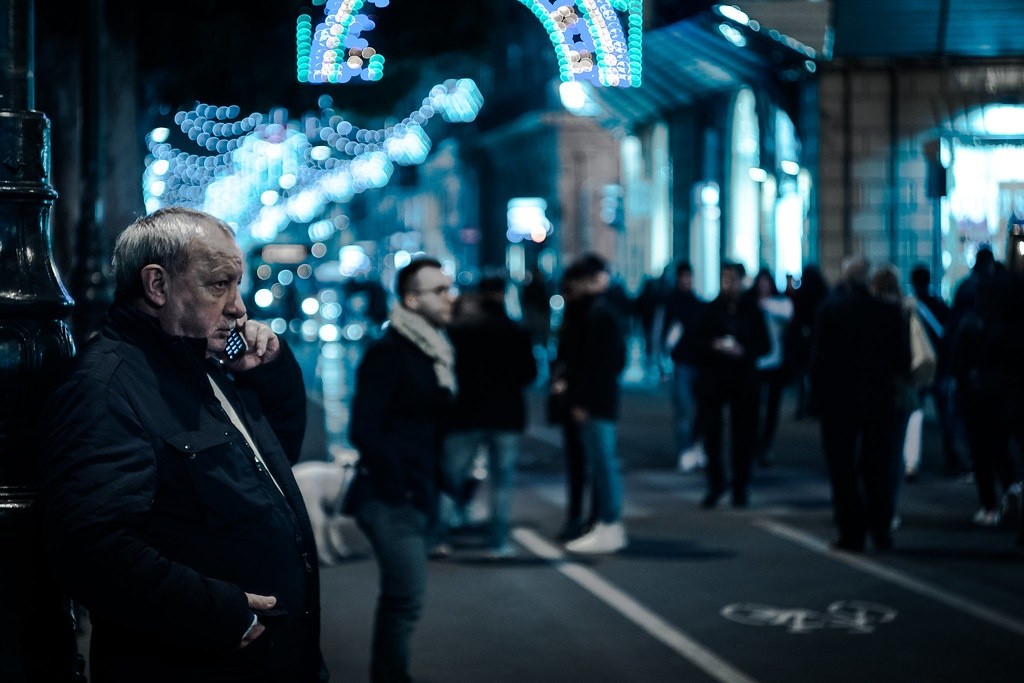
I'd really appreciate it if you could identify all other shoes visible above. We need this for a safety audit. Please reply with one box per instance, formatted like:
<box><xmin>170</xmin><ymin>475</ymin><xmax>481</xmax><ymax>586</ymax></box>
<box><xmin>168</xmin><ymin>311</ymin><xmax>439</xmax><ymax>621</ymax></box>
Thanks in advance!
<box><xmin>732</xmin><ymin>480</ymin><xmax>749</xmax><ymax>505</ymax></box>
<box><xmin>428</xmin><ymin>541</ymin><xmax>454</xmax><ymax>559</ymax></box>
<box><xmin>557</xmin><ymin>518</ymin><xmax>592</xmax><ymax>542</ymax></box>
<box><xmin>871</xmin><ymin>532</ymin><xmax>892</xmax><ymax>550</ymax></box>
<box><xmin>702</xmin><ymin>478</ymin><xmax>729</xmax><ymax>507</ymax></box>
<box><xmin>973</xmin><ymin>507</ymin><xmax>1001</xmax><ymax>527</ymax></box>
<box><xmin>481</xmin><ymin>542</ymin><xmax>518</xmax><ymax>561</ymax></box>
<box><xmin>678</xmin><ymin>446</ymin><xmax>708</xmax><ymax>474</ymax></box>
<box><xmin>828</xmin><ymin>535</ymin><xmax>867</xmax><ymax>553</ymax></box>
<box><xmin>564</xmin><ymin>519</ymin><xmax>629</xmax><ymax>556</ymax></box>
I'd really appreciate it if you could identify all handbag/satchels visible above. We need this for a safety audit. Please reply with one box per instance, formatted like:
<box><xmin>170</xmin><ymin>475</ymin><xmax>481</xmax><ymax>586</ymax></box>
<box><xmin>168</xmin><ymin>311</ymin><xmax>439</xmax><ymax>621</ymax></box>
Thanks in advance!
<box><xmin>904</xmin><ymin>293</ymin><xmax>938</xmax><ymax>386</ymax></box>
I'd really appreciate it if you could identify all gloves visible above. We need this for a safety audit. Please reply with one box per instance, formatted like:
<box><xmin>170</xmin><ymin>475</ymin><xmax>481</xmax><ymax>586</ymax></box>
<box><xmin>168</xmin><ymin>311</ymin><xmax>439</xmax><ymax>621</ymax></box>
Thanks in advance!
<box><xmin>436</xmin><ymin>464</ymin><xmax>477</xmax><ymax>508</ymax></box>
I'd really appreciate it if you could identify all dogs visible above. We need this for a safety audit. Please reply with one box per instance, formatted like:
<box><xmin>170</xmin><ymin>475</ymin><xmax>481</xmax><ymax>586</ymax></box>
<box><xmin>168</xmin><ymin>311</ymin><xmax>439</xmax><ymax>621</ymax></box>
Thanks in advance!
<box><xmin>291</xmin><ymin>460</ymin><xmax>357</xmax><ymax>569</ymax></box>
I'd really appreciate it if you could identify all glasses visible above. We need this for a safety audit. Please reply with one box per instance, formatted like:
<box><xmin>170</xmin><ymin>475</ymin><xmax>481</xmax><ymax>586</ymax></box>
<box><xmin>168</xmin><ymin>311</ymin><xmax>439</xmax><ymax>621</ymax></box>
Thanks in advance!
<box><xmin>415</xmin><ymin>284</ymin><xmax>449</xmax><ymax>295</ymax></box>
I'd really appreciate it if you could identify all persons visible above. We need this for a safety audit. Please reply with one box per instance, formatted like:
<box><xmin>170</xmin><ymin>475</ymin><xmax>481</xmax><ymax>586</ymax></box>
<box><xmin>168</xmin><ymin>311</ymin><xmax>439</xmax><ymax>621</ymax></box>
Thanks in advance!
<box><xmin>343</xmin><ymin>243</ymin><xmax>1024</xmax><ymax>683</ymax></box>
<box><xmin>46</xmin><ymin>207</ymin><xmax>327</xmax><ymax>683</ymax></box>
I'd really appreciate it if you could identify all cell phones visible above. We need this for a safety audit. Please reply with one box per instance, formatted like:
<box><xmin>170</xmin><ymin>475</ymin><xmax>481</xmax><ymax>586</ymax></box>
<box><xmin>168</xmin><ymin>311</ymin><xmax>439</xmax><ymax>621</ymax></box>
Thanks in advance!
<box><xmin>224</xmin><ymin>327</ymin><xmax>249</xmax><ymax>361</ymax></box>
